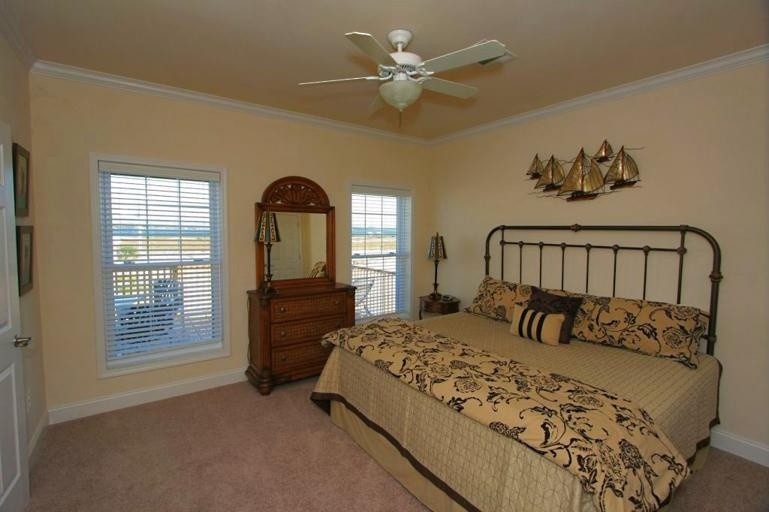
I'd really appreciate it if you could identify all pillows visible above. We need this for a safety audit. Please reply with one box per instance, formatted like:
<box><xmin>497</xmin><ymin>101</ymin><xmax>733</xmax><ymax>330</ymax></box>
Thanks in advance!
<box><xmin>463</xmin><ymin>275</ymin><xmax>712</xmax><ymax>369</ymax></box>
<box><xmin>308</xmin><ymin>261</ymin><xmax>326</xmax><ymax>278</ymax></box>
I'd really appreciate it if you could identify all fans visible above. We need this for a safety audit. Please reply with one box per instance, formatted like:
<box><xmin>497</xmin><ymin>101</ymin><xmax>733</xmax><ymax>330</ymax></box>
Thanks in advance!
<box><xmin>298</xmin><ymin>29</ymin><xmax>505</xmax><ymax>105</ymax></box>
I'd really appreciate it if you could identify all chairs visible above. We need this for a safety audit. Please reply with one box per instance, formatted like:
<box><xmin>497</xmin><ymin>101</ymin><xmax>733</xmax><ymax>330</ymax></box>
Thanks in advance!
<box><xmin>114</xmin><ymin>279</ymin><xmax>184</xmax><ymax>358</ymax></box>
<box><xmin>351</xmin><ymin>280</ymin><xmax>374</xmax><ymax>320</ymax></box>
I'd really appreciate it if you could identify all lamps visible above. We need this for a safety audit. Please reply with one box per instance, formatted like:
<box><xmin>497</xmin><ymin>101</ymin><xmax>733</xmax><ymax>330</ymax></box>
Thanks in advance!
<box><xmin>378</xmin><ymin>72</ymin><xmax>423</xmax><ymax>113</ymax></box>
<box><xmin>427</xmin><ymin>231</ymin><xmax>446</xmax><ymax>300</ymax></box>
<box><xmin>255</xmin><ymin>211</ymin><xmax>281</xmax><ymax>297</ymax></box>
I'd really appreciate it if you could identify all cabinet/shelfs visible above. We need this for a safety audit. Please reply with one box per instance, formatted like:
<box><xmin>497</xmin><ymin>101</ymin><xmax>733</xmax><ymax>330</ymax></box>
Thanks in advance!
<box><xmin>247</xmin><ymin>287</ymin><xmax>357</xmax><ymax>396</ymax></box>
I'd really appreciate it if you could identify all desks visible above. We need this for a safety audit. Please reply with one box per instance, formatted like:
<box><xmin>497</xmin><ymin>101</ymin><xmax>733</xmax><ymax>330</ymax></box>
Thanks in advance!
<box><xmin>419</xmin><ymin>294</ymin><xmax>459</xmax><ymax>321</ymax></box>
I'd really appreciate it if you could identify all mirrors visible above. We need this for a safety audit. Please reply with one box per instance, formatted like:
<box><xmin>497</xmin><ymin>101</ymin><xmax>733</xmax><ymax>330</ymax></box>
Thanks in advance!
<box><xmin>255</xmin><ymin>176</ymin><xmax>335</xmax><ymax>291</ymax></box>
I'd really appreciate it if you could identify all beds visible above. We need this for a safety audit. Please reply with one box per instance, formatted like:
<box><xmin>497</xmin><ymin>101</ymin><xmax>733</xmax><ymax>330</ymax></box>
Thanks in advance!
<box><xmin>310</xmin><ymin>224</ymin><xmax>720</xmax><ymax>512</ymax></box>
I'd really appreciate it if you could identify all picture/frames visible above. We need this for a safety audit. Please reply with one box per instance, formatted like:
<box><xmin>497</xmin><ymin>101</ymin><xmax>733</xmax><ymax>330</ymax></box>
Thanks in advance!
<box><xmin>16</xmin><ymin>225</ymin><xmax>33</xmax><ymax>296</ymax></box>
<box><xmin>12</xmin><ymin>142</ymin><xmax>30</xmax><ymax>216</ymax></box>
